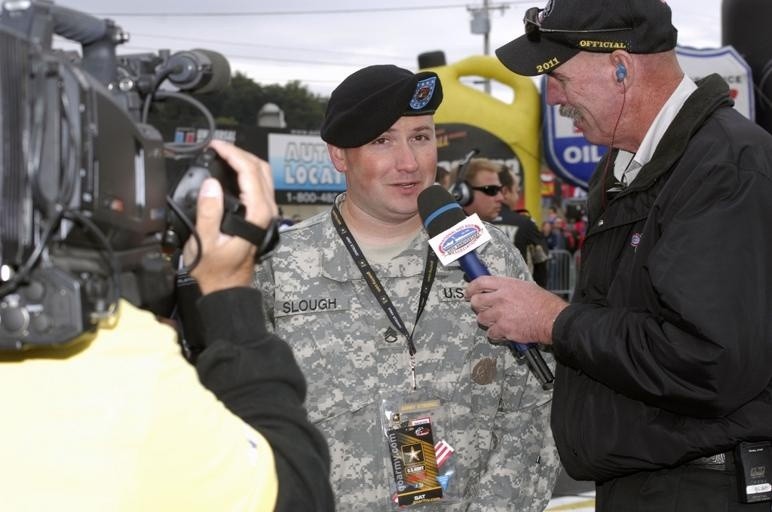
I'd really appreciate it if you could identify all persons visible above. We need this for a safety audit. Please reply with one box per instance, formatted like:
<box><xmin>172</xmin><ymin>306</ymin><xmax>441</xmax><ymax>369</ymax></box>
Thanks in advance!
<box><xmin>462</xmin><ymin>1</ymin><xmax>771</xmax><ymax>510</ymax></box>
<box><xmin>437</xmin><ymin>158</ymin><xmax>585</xmax><ymax>287</ymax></box>
<box><xmin>251</xmin><ymin>64</ymin><xmax>563</xmax><ymax>512</ymax></box>
<box><xmin>1</xmin><ymin>139</ymin><xmax>337</xmax><ymax>510</ymax></box>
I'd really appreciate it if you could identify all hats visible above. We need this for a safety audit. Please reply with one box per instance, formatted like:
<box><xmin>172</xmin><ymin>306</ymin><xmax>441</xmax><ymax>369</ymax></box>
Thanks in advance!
<box><xmin>496</xmin><ymin>1</ymin><xmax>677</xmax><ymax>76</ymax></box>
<box><xmin>320</xmin><ymin>65</ymin><xmax>443</xmax><ymax>148</ymax></box>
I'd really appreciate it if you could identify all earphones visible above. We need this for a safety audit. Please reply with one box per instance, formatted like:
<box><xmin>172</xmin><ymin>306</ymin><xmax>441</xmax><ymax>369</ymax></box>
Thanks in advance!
<box><xmin>616</xmin><ymin>64</ymin><xmax>626</xmax><ymax>81</ymax></box>
<box><xmin>448</xmin><ymin>181</ymin><xmax>473</xmax><ymax>207</ymax></box>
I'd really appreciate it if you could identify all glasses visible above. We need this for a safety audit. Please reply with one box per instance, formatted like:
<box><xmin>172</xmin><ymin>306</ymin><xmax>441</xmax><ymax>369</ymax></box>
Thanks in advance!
<box><xmin>472</xmin><ymin>185</ymin><xmax>503</xmax><ymax>196</ymax></box>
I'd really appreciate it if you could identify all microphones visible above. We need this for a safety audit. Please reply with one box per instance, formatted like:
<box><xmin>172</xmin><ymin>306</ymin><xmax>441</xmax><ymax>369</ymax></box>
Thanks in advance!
<box><xmin>456</xmin><ymin>148</ymin><xmax>480</xmax><ymax>181</ymax></box>
<box><xmin>417</xmin><ymin>185</ymin><xmax>555</xmax><ymax>387</ymax></box>
